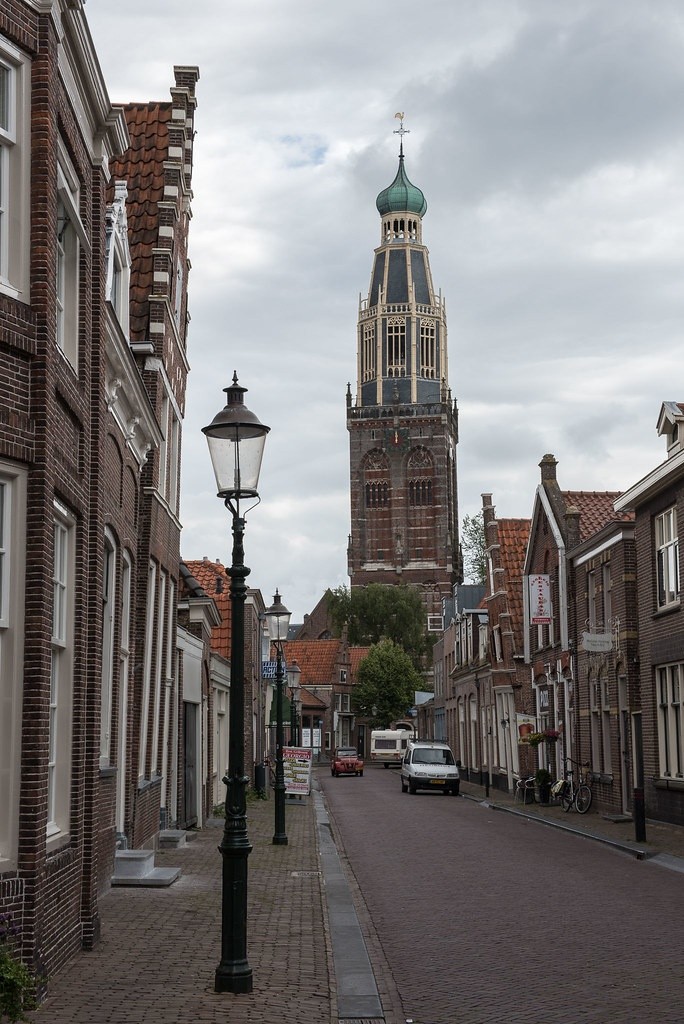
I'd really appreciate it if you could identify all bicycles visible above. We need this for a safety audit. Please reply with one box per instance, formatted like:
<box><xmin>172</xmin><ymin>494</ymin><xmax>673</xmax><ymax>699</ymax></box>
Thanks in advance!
<box><xmin>559</xmin><ymin>757</ymin><xmax>593</xmax><ymax>813</ymax></box>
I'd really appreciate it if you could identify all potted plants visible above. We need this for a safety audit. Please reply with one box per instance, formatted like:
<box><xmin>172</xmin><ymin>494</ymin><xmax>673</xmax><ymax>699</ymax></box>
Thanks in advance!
<box><xmin>526</xmin><ymin>732</ymin><xmax>544</xmax><ymax>751</ymax></box>
<box><xmin>518</xmin><ymin>768</ymin><xmax>535</xmax><ymax>804</ymax></box>
<box><xmin>535</xmin><ymin>769</ymin><xmax>553</xmax><ymax>803</ymax></box>
<box><xmin>540</xmin><ymin>729</ymin><xmax>561</xmax><ymax>742</ymax></box>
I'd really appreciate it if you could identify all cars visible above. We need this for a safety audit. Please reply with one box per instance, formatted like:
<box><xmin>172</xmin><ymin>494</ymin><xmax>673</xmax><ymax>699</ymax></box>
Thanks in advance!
<box><xmin>401</xmin><ymin>735</ymin><xmax>460</xmax><ymax>796</ymax></box>
<box><xmin>330</xmin><ymin>746</ymin><xmax>364</xmax><ymax>777</ymax></box>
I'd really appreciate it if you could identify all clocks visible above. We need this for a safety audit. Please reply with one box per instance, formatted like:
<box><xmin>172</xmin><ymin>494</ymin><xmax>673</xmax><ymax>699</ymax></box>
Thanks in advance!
<box><xmin>384</xmin><ymin>427</ymin><xmax>411</xmax><ymax>453</ymax></box>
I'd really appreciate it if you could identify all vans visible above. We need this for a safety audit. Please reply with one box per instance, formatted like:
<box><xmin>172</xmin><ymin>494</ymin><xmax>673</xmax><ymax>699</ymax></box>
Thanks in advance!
<box><xmin>370</xmin><ymin>729</ymin><xmax>418</xmax><ymax>770</ymax></box>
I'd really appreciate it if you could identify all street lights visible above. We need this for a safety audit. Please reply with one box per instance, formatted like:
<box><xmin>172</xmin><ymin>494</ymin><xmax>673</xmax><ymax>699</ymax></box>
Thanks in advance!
<box><xmin>201</xmin><ymin>368</ymin><xmax>271</xmax><ymax>996</ymax></box>
<box><xmin>264</xmin><ymin>589</ymin><xmax>293</xmax><ymax>846</ymax></box>
<box><xmin>285</xmin><ymin>659</ymin><xmax>301</xmax><ymax>745</ymax></box>
